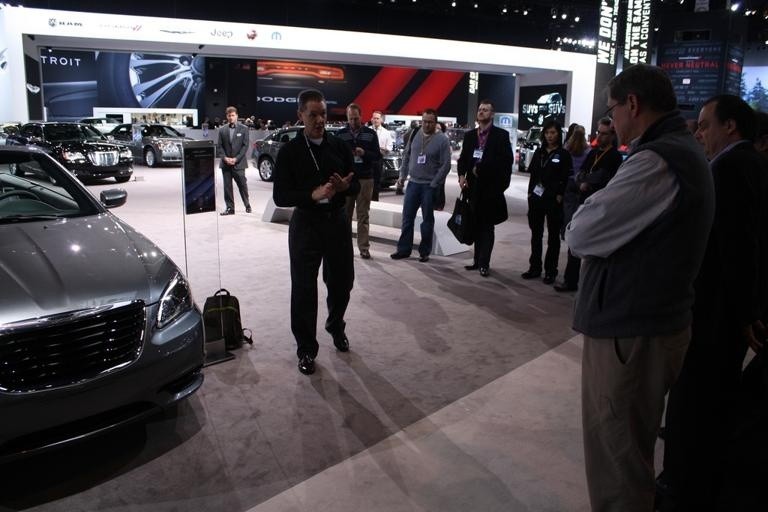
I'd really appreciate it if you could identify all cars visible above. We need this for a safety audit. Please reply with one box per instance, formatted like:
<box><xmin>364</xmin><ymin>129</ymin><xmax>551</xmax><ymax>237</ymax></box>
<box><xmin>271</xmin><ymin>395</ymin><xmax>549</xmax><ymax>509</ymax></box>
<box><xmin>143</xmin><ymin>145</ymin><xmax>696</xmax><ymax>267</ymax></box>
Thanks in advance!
<box><xmin>518</xmin><ymin>128</ymin><xmax>572</xmax><ymax>170</ymax></box>
<box><xmin>106</xmin><ymin>123</ymin><xmax>201</xmax><ymax>166</ymax></box>
<box><xmin>6</xmin><ymin>123</ymin><xmax>134</xmax><ymax>184</ymax></box>
<box><xmin>1</xmin><ymin>144</ymin><xmax>206</xmax><ymax>447</ymax></box>
<box><xmin>251</xmin><ymin>127</ymin><xmax>400</xmax><ymax>186</ymax></box>
<box><xmin>442</xmin><ymin>128</ymin><xmax>471</xmax><ymax>147</ymax></box>
<box><xmin>1</xmin><ymin>127</ymin><xmax>19</xmax><ymax>151</ymax></box>
<box><xmin>77</xmin><ymin>117</ymin><xmax>123</xmax><ymax>138</ymax></box>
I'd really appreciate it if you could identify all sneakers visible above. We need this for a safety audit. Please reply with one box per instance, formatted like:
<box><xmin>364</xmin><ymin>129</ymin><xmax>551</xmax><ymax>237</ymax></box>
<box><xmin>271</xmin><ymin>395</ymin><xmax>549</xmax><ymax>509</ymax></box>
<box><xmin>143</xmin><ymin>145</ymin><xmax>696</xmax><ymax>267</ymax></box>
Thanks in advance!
<box><xmin>543</xmin><ymin>272</ymin><xmax>556</xmax><ymax>284</ymax></box>
<box><xmin>522</xmin><ymin>268</ymin><xmax>541</xmax><ymax>279</ymax></box>
<box><xmin>554</xmin><ymin>282</ymin><xmax>575</xmax><ymax>293</ymax></box>
<box><xmin>360</xmin><ymin>250</ymin><xmax>370</xmax><ymax>258</ymax></box>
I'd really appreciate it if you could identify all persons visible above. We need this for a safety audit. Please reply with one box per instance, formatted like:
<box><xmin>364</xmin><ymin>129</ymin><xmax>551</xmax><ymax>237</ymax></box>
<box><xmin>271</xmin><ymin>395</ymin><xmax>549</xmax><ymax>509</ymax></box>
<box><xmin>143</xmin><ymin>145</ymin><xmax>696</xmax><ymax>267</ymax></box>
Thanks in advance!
<box><xmin>740</xmin><ymin>113</ymin><xmax>768</xmax><ymax>159</ymax></box>
<box><xmin>564</xmin><ymin>64</ymin><xmax>716</xmax><ymax>512</ymax></box>
<box><xmin>273</xmin><ymin>89</ymin><xmax>361</xmax><ymax>375</ymax></box>
<box><xmin>217</xmin><ymin>106</ymin><xmax>252</xmax><ymax>215</ymax></box>
<box><xmin>432</xmin><ymin>122</ymin><xmax>446</xmax><ymax>210</ymax></box>
<box><xmin>390</xmin><ymin>109</ymin><xmax>451</xmax><ymax>262</ymax></box>
<box><xmin>522</xmin><ymin>120</ymin><xmax>573</xmax><ymax>285</ymax></box>
<box><xmin>200</xmin><ymin>115</ymin><xmax>277</xmax><ymax>130</ymax></box>
<box><xmin>563</xmin><ymin>127</ymin><xmax>591</xmax><ymax>240</ymax></box>
<box><xmin>564</xmin><ymin>124</ymin><xmax>577</xmax><ymax>144</ymax></box>
<box><xmin>653</xmin><ymin>95</ymin><xmax>768</xmax><ymax>512</ymax></box>
<box><xmin>458</xmin><ymin>100</ymin><xmax>514</xmax><ymax>277</ymax></box>
<box><xmin>334</xmin><ymin>103</ymin><xmax>380</xmax><ymax>258</ymax></box>
<box><xmin>554</xmin><ymin>117</ymin><xmax>623</xmax><ymax>291</ymax></box>
<box><xmin>367</xmin><ymin>111</ymin><xmax>393</xmax><ymax>201</ymax></box>
<box><xmin>396</xmin><ymin>120</ymin><xmax>419</xmax><ymax>195</ymax></box>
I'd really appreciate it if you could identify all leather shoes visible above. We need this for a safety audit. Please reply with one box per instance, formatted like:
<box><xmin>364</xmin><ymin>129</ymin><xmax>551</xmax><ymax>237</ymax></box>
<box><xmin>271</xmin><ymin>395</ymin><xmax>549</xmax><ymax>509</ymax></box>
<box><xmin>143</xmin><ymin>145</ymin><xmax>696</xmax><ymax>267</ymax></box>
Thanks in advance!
<box><xmin>480</xmin><ymin>267</ymin><xmax>489</xmax><ymax>277</ymax></box>
<box><xmin>464</xmin><ymin>264</ymin><xmax>477</xmax><ymax>270</ymax></box>
<box><xmin>246</xmin><ymin>206</ymin><xmax>251</xmax><ymax>212</ymax></box>
<box><xmin>298</xmin><ymin>352</ymin><xmax>316</xmax><ymax>375</ymax></box>
<box><xmin>419</xmin><ymin>255</ymin><xmax>428</xmax><ymax>261</ymax></box>
<box><xmin>220</xmin><ymin>208</ymin><xmax>235</xmax><ymax>215</ymax></box>
<box><xmin>332</xmin><ymin>333</ymin><xmax>348</xmax><ymax>352</ymax></box>
<box><xmin>391</xmin><ymin>249</ymin><xmax>410</xmax><ymax>259</ymax></box>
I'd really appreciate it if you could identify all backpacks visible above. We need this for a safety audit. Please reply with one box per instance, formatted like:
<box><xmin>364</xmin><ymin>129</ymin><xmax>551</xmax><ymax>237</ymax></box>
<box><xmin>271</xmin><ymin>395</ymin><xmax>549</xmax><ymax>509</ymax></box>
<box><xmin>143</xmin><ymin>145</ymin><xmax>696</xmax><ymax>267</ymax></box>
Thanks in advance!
<box><xmin>203</xmin><ymin>289</ymin><xmax>253</xmax><ymax>351</ymax></box>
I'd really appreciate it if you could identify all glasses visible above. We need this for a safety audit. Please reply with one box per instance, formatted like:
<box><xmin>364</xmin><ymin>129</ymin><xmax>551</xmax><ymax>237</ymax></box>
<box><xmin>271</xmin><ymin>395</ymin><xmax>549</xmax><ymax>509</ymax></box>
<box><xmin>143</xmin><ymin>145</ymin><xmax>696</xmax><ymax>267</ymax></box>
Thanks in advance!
<box><xmin>606</xmin><ymin>101</ymin><xmax>621</xmax><ymax>122</ymax></box>
<box><xmin>595</xmin><ymin>130</ymin><xmax>608</xmax><ymax>136</ymax></box>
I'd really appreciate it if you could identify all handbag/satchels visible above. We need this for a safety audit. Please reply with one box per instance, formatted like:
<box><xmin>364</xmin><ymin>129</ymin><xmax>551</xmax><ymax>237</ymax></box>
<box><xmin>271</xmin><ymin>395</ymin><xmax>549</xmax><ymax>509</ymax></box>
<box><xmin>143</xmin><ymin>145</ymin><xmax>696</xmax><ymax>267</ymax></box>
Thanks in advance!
<box><xmin>433</xmin><ymin>184</ymin><xmax>445</xmax><ymax>210</ymax></box>
<box><xmin>446</xmin><ymin>186</ymin><xmax>474</xmax><ymax>245</ymax></box>
